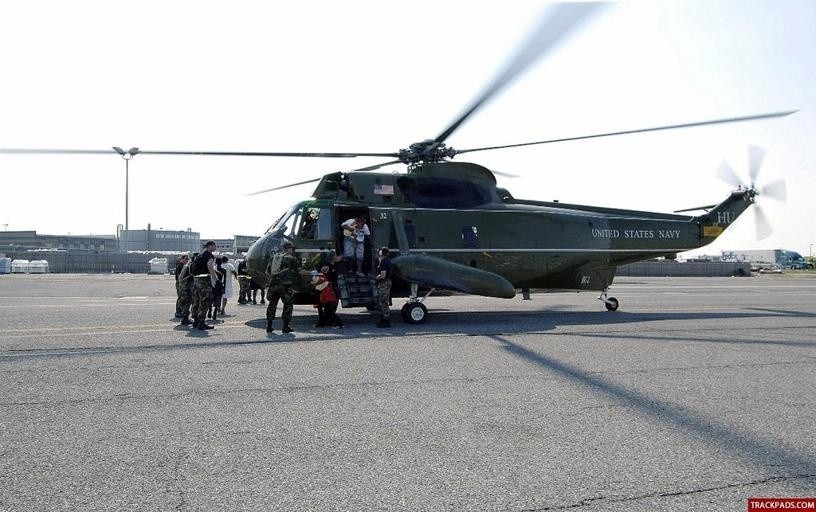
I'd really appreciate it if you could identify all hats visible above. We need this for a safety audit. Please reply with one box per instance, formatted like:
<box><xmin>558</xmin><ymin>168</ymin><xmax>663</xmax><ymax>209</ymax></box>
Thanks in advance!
<box><xmin>327</xmin><ymin>250</ymin><xmax>336</xmax><ymax>260</ymax></box>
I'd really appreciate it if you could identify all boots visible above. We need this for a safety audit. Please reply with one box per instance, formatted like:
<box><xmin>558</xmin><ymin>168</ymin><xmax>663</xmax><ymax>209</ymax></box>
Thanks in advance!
<box><xmin>266</xmin><ymin>318</ymin><xmax>295</xmax><ymax>333</ymax></box>
<box><xmin>376</xmin><ymin>315</ymin><xmax>390</xmax><ymax>328</ymax></box>
<box><xmin>173</xmin><ymin>307</ymin><xmax>224</xmax><ymax>331</ymax></box>
<box><xmin>236</xmin><ymin>295</ymin><xmax>269</xmax><ymax>306</ymax></box>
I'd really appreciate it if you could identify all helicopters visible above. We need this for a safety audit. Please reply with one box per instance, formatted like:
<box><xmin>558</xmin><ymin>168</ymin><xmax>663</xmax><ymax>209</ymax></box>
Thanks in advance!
<box><xmin>0</xmin><ymin>3</ymin><xmax>802</xmax><ymax>325</ymax></box>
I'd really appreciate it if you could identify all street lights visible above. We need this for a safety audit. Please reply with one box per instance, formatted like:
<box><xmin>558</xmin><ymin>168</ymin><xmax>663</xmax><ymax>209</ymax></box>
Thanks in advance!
<box><xmin>112</xmin><ymin>147</ymin><xmax>141</xmax><ymax>274</ymax></box>
<box><xmin>115</xmin><ymin>222</ymin><xmax>124</xmax><ymax>271</ymax></box>
<box><xmin>807</xmin><ymin>243</ymin><xmax>815</xmax><ymax>257</ymax></box>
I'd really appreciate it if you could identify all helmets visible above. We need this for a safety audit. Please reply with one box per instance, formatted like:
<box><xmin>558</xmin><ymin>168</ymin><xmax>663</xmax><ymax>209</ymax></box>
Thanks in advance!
<box><xmin>377</xmin><ymin>247</ymin><xmax>390</xmax><ymax>256</ymax></box>
<box><xmin>282</xmin><ymin>241</ymin><xmax>296</xmax><ymax>248</ymax></box>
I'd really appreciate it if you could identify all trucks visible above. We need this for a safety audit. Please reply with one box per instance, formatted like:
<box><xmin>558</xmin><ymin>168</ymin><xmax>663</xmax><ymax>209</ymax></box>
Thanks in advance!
<box><xmin>721</xmin><ymin>246</ymin><xmax>806</xmax><ymax>270</ymax></box>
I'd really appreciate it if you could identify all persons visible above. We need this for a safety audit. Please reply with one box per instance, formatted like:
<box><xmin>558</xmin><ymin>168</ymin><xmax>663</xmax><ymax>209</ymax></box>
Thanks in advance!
<box><xmin>266</xmin><ymin>243</ymin><xmax>303</xmax><ymax>334</ymax></box>
<box><xmin>340</xmin><ymin>215</ymin><xmax>371</xmax><ymax>280</ymax></box>
<box><xmin>172</xmin><ymin>239</ymin><xmax>265</xmax><ymax>331</ymax></box>
<box><xmin>308</xmin><ymin>248</ymin><xmax>346</xmax><ymax>331</ymax></box>
<box><xmin>375</xmin><ymin>248</ymin><xmax>394</xmax><ymax>328</ymax></box>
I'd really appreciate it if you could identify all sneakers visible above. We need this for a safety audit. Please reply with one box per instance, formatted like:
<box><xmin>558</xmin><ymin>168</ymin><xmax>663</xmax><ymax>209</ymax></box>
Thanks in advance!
<box><xmin>355</xmin><ymin>270</ymin><xmax>366</xmax><ymax>277</ymax></box>
<box><xmin>312</xmin><ymin>319</ymin><xmax>347</xmax><ymax>331</ymax></box>
